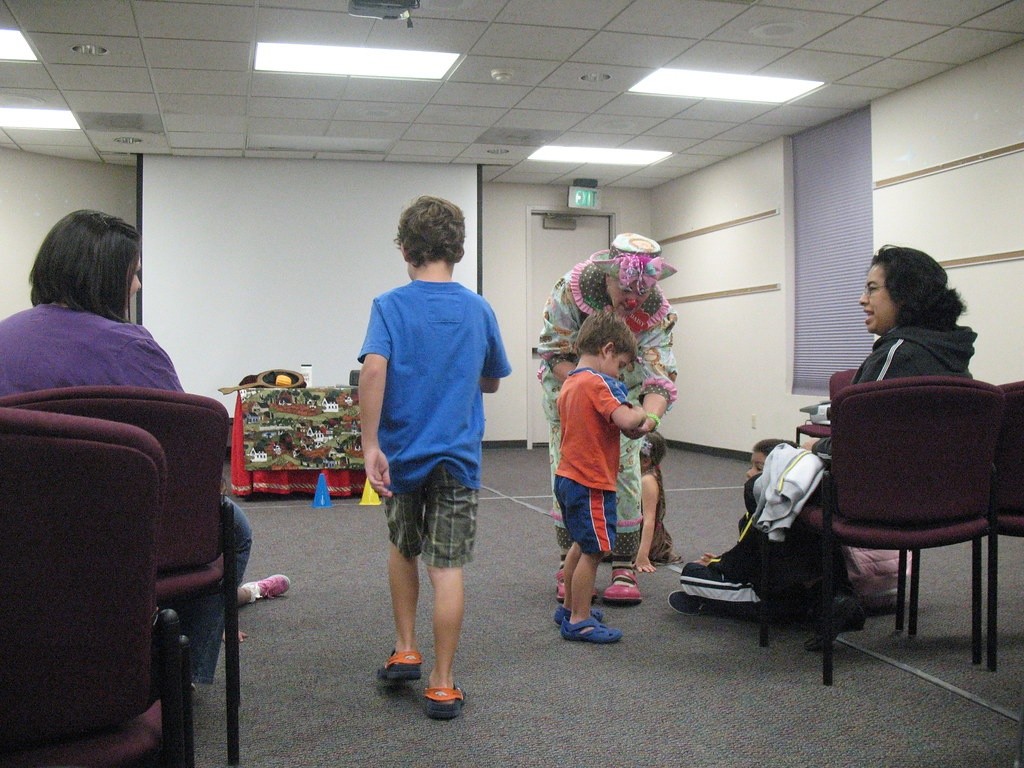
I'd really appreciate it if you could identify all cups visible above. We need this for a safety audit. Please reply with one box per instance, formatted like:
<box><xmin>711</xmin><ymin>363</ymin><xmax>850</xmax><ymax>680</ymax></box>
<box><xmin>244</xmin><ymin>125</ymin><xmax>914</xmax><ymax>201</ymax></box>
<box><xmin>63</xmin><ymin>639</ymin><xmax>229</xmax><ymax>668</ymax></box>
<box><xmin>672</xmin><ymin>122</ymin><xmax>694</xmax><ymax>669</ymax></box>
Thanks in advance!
<box><xmin>301</xmin><ymin>365</ymin><xmax>312</xmax><ymax>387</ymax></box>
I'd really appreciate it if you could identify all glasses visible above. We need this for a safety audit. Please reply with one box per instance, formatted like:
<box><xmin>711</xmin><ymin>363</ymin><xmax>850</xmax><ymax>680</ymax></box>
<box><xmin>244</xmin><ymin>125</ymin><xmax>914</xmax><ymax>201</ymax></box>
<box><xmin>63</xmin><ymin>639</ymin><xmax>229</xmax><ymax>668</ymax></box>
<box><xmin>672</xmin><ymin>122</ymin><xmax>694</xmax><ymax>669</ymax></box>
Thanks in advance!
<box><xmin>864</xmin><ymin>283</ymin><xmax>886</xmax><ymax>297</ymax></box>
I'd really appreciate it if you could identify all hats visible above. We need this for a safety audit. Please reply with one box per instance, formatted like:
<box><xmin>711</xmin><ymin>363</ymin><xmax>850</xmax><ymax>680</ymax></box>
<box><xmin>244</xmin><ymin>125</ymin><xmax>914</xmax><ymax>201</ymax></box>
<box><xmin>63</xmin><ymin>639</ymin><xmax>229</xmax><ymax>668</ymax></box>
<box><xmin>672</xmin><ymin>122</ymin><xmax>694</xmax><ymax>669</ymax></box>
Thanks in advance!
<box><xmin>590</xmin><ymin>232</ymin><xmax>678</xmax><ymax>296</ymax></box>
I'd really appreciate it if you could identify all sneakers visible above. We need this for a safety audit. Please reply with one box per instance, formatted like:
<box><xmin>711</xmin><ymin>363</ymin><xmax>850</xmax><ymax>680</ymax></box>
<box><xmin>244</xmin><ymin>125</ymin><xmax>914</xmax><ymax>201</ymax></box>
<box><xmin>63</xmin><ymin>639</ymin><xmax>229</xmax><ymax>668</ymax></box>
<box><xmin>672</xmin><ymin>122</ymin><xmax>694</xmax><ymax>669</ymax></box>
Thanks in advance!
<box><xmin>242</xmin><ymin>574</ymin><xmax>290</xmax><ymax>604</ymax></box>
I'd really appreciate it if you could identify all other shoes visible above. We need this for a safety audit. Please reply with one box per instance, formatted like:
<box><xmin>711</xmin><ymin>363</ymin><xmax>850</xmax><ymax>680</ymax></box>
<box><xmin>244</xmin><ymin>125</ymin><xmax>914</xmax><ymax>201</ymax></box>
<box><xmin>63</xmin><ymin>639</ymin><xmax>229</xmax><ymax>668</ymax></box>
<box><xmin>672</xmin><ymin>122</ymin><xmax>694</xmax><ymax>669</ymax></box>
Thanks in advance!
<box><xmin>803</xmin><ymin>588</ymin><xmax>865</xmax><ymax>652</ymax></box>
<box><xmin>667</xmin><ymin>590</ymin><xmax>714</xmax><ymax>616</ymax></box>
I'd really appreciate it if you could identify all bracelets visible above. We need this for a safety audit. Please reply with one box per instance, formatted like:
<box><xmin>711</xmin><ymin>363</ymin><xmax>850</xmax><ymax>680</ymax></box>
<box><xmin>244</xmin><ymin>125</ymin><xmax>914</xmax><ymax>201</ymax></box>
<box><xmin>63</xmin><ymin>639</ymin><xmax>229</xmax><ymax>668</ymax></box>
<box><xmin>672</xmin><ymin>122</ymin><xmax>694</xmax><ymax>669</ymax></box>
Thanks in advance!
<box><xmin>646</xmin><ymin>413</ymin><xmax>661</xmax><ymax>434</ymax></box>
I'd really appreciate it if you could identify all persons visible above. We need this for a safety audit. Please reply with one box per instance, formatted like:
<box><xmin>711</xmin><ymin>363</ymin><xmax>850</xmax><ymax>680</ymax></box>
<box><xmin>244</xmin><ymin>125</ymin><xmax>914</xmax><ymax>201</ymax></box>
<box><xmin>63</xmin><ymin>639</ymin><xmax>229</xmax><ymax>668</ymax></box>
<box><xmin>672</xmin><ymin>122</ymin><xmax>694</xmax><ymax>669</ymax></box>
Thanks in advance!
<box><xmin>668</xmin><ymin>440</ymin><xmax>798</xmax><ymax>619</ymax></box>
<box><xmin>358</xmin><ymin>194</ymin><xmax>513</xmax><ymax>719</ymax></box>
<box><xmin>222</xmin><ymin>574</ymin><xmax>291</xmax><ymax>644</ymax></box>
<box><xmin>537</xmin><ymin>232</ymin><xmax>683</xmax><ymax>644</ymax></box>
<box><xmin>788</xmin><ymin>245</ymin><xmax>978</xmax><ymax>651</ymax></box>
<box><xmin>0</xmin><ymin>210</ymin><xmax>253</xmax><ymax>705</ymax></box>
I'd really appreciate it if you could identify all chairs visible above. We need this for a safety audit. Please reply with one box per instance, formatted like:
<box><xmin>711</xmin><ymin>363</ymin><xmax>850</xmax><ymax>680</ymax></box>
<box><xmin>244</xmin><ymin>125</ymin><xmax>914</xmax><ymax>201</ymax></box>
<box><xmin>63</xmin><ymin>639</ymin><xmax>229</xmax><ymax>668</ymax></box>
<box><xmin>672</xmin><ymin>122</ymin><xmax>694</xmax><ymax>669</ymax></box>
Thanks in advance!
<box><xmin>759</xmin><ymin>369</ymin><xmax>1024</xmax><ymax>684</ymax></box>
<box><xmin>1</xmin><ymin>383</ymin><xmax>242</xmax><ymax>767</ymax></box>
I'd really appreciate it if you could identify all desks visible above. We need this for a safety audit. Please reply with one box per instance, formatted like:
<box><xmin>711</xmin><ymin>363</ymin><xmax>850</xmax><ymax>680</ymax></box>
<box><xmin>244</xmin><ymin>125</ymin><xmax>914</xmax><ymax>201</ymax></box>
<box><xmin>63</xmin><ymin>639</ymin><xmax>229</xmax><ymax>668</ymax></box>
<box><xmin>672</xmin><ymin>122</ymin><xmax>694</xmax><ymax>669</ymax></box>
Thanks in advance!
<box><xmin>232</xmin><ymin>386</ymin><xmax>376</xmax><ymax>497</ymax></box>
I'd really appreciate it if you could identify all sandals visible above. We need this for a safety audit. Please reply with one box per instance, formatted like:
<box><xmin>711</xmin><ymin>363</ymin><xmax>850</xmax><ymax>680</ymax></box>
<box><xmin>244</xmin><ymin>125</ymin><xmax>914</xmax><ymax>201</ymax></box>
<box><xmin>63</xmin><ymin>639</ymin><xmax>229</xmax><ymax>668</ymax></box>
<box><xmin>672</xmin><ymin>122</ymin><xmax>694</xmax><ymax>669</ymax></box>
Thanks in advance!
<box><xmin>560</xmin><ymin>615</ymin><xmax>623</xmax><ymax>644</ymax></box>
<box><xmin>554</xmin><ymin>605</ymin><xmax>604</xmax><ymax>625</ymax></box>
<box><xmin>420</xmin><ymin>682</ymin><xmax>467</xmax><ymax>721</ymax></box>
<box><xmin>376</xmin><ymin>648</ymin><xmax>423</xmax><ymax>681</ymax></box>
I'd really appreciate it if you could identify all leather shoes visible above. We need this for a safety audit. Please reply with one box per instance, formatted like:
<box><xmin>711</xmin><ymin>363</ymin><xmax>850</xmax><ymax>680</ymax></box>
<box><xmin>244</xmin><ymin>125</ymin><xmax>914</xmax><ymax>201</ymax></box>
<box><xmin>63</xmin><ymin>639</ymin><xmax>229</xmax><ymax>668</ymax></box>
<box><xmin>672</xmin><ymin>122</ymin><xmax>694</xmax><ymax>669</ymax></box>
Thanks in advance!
<box><xmin>602</xmin><ymin>571</ymin><xmax>642</xmax><ymax>604</ymax></box>
<box><xmin>555</xmin><ymin>567</ymin><xmax>599</xmax><ymax>602</ymax></box>
